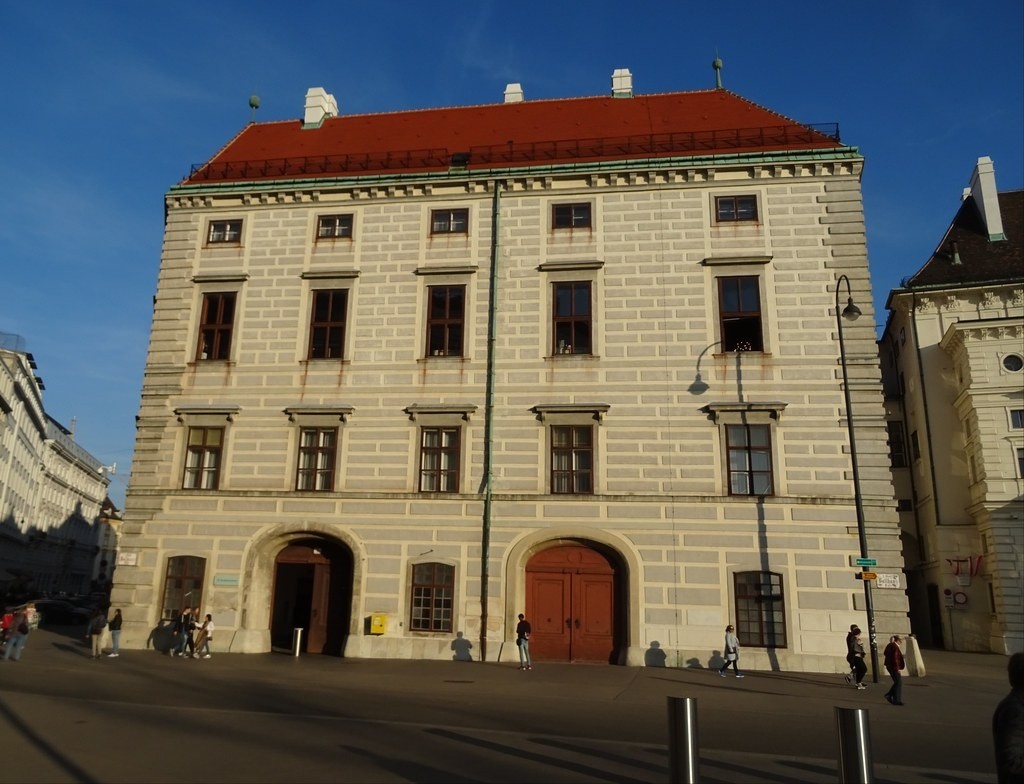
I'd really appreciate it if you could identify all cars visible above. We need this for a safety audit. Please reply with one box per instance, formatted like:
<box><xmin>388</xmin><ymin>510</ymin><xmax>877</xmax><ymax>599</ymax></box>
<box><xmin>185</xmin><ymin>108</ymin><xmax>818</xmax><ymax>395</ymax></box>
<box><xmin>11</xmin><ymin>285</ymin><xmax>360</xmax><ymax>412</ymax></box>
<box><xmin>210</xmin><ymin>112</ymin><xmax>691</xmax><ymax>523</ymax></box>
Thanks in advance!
<box><xmin>4</xmin><ymin>600</ymin><xmax>93</xmax><ymax>629</ymax></box>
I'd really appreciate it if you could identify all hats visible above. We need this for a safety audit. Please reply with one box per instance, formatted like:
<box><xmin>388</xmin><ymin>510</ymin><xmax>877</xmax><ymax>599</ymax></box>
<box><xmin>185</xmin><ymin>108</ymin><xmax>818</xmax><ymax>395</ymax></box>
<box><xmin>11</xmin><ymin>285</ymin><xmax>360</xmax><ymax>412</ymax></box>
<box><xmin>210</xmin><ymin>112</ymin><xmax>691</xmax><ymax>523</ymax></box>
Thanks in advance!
<box><xmin>853</xmin><ymin>627</ymin><xmax>860</xmax><ymax>634</ymax></box>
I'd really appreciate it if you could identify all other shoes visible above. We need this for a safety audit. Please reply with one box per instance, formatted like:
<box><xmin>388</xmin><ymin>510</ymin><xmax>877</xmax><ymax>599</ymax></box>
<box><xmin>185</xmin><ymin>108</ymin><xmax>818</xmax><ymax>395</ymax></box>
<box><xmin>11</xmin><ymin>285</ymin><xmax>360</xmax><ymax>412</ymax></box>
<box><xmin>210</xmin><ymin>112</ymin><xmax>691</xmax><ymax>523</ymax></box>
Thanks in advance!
<box><xmin>170</xmin><ymin>648</ymin><xmax>174</xmax><ymax>657</ymax></box>
<box><xmin>193</xmin><ymin>653</ymin><xmax>199</xmax><ymax>658</ymax></box>
<box><xmin>855</xmin><ymin>682</ymin><xmax>868</xmax><ymax>687</ymax></box>
<box><xmin>893</xmin><ymin>701</ymin><xmax>904</xmax><ymax>705</ymax></box>
<box><xmin>184</xmin><ymin>654</ymin><xmax>188</xmax><ymax>658</ymax></box>
<box><xmin>108</xmin><ymin>653</ymin><xmax>119</xmax><ymax>657</ymax></box>
<box><xmin>203</xmin><ymin>655</ymin><xmax>211</xmax><ymax>658</ymax></box>
<box><xmin>884</xmin><ymin>694</ymin><xmax>893</xmax><ymax>704</ymax></box>
<box><xmin>858</xmin><ymin>683</ymin><xmax>866</xmax><ymax>690</ymax></box>
<box><xmin>89</xmin><ymin>655</ymin><xmax>102</xmax><ymax>659</ymax></box>
<box><xmin>845</xmin><ymin>676</ymin><xmax>851</xmax><ymax>685</ymax></box>
<box><xmin>519</xmin><ymin>666</ymin><xmax>526</xmax><ymax>670</ymax></box>
<box><xmin>527</xmin><ymin>666</ymin><xmax>531</xmax><ymax>669</ymax></box>
<box><xmin>175</xmin><ymin>652</ymin><xmax>183</xmax><ymax>656</ymax></box>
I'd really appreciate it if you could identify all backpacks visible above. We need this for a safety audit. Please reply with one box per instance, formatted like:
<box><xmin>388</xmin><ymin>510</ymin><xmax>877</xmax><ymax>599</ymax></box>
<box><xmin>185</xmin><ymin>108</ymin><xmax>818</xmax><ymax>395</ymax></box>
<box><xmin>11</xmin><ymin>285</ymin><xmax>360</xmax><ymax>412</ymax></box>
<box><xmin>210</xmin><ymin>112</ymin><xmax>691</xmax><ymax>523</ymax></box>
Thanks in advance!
<box><xmin>91</xmin><ymin>620</ymin><xmax>101</xmax><ymax>634</ymax></box>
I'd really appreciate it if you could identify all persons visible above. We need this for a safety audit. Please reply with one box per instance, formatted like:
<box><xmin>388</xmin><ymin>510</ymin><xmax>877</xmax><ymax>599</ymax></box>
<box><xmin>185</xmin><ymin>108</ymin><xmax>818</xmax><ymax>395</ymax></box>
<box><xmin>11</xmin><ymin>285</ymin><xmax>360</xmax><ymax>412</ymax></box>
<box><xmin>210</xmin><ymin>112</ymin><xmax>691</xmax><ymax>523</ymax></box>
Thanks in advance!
<box><xmin>845</xmin><ymin>624</ymin><xmax>868</xmax><ymax>689</ymax></box>
<box><xmin>883</xmin><ymin>634</ymin><xmax>905</xmax><ymax>706</ymax></box>
<box><xmin>169</xmin><ymin>606</ymin><xmax>214</xmax><ymax>659</ymax></box>
<box><xmin>718</xmin><ymin>625</ymin><xmax>744</xmax><ymax>677</ymax></box>
<box><xmin>515</xmin><ymin>614</ymin><xmax>532</xmax><ymax>670</ymax></box>
<box><xmin>86</xmin><ymin>606</ymin><xmax>122</xmax><ymax>658</ymax></box>
<box><xmin>0</xmin><ymin>600</ymin><xmax>36</xmax><ymax>661</ymax></box>
<box><xmin>992</xmin><ymin>652</ymin><xmax>1024</xmax><ymax>784</ymax></box>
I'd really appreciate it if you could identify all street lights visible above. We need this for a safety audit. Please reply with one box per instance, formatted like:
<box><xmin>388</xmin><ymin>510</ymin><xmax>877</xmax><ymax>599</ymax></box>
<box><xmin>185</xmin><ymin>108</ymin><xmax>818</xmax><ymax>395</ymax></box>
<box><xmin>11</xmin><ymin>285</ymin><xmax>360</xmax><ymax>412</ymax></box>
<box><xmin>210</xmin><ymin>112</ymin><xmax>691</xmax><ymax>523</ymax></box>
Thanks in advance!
<box><xmin>833</xmin><ymin>274</ymin><xmax>879</xmax><ymax>684</ymax></box>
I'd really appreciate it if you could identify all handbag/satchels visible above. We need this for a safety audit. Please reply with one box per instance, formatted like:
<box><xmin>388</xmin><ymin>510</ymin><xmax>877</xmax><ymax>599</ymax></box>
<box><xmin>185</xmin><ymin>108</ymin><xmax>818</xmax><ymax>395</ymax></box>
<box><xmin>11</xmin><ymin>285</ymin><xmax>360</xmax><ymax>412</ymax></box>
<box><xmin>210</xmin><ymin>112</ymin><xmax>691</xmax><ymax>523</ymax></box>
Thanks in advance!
<box><xmin>17</xmin><ymin>617</ymin><xmax>29</xmax><ymax>635</ymax></box>
<box><xmin>517</xmin><ymin>639</ymin><xmax>521</xmax><ymax>646</ymax></box>
<box><xmin>727</xmin><ymin>647</ymin><xmax>736</xmax><ymax>654</ymax></box>
<box><xmin>853</xmin><ymin>639</ymin><xmax>862</xmax><ymax>653</ymax></box>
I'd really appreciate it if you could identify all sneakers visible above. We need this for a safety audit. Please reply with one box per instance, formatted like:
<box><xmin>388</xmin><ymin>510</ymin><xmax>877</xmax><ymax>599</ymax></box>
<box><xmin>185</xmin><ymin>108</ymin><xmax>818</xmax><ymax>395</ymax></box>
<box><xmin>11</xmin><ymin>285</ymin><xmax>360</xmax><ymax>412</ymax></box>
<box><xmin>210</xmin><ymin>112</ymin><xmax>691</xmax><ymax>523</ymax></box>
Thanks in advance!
<box><xmin>736</xmin><ymin>674</ymin><xmax>744</xmax><ymax>677</ymax></box>
<box><xmin>719</xmin><ymin>670</ymin><xmax>726</xmax><ymax>677</ymax></box>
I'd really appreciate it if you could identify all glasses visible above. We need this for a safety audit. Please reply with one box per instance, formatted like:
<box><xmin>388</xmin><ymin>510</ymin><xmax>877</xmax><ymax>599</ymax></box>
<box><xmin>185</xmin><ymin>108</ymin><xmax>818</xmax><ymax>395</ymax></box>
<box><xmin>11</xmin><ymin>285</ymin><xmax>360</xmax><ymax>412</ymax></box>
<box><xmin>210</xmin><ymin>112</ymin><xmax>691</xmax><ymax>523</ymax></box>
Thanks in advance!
<box><xmin>729</xmin><ymin>628</ymin><xmax>733</xmax><ymax>630</ymax></box>
